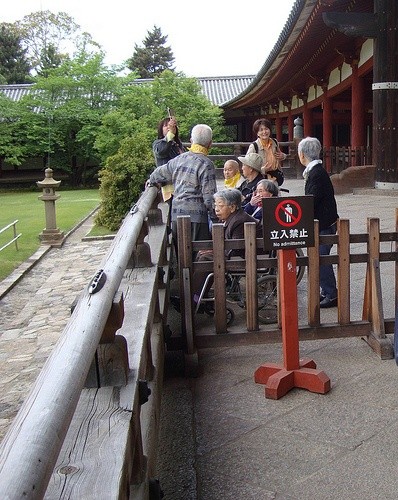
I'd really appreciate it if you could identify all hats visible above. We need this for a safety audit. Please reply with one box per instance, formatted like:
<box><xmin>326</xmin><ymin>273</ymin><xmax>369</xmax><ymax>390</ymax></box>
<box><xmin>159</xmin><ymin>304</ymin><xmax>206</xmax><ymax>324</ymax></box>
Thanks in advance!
<box><xmin>238</xmin><ymin>153</ymin><xmax>263</xmax><ymax>173</ymax></box>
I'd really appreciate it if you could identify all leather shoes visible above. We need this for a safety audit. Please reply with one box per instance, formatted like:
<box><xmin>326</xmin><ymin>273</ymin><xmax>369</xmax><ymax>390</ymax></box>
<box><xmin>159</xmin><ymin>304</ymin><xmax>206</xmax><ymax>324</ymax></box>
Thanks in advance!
<box><xmin>320</xmin><ymin>293</ymin><xmax>338</xmax><ymax>308</ymax></box>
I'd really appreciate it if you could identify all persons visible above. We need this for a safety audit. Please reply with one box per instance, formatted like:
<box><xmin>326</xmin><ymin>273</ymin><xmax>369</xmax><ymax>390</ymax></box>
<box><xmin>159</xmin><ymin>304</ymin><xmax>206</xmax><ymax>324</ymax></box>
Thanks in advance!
<box><xmin>190</xmin><ymin>188</ymin><xmax>265</xmax><ymax>311</ymax></box>
<box><xmin>236</xmin><ymin>155</ymin><xmax>270</xmax><ymax>193</ymax></box>
<box><xmin>247</xmin><ymin>118</ymin><xmax>290</xmax><ymax>187</ymax></box>
<box><xmin>145</xmin><ymin>123</ymin><xmax>218</xmax><ymax>263</ymax></box>
<box><xmin>153</xmin><ymin>116</ymin><xmax>189</xmax><ymax>221</ymax></box>
<box><xmin>295</xmin><ymin>135</ymin><xmax>346</xmax><ymax>307</ymax></box>
<box><xmin>218</xmin><ymin>157</ymin><xmax>248</xmax><ymax>192</ymax></box>
<box><xmin>243</xmin><ymin>179</ymin><xmax>284</xmax><ymax>220</ymax></box>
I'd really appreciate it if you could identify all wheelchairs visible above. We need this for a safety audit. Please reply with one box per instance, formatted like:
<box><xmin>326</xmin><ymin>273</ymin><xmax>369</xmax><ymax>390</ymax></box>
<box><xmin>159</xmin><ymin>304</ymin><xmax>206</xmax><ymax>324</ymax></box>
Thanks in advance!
<box><xmin>167</xmin><ymin>188</ymin><xmax>306</xmax><ymax>330</ymax></box>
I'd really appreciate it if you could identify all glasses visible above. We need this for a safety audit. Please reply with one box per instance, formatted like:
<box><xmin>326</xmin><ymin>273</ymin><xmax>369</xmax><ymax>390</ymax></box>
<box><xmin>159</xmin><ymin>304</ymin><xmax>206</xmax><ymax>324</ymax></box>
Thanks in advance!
<box><xmin>212</xmin><ymin>203</ymin><xmax>228</xmax><ymax>209</ymax></box>
